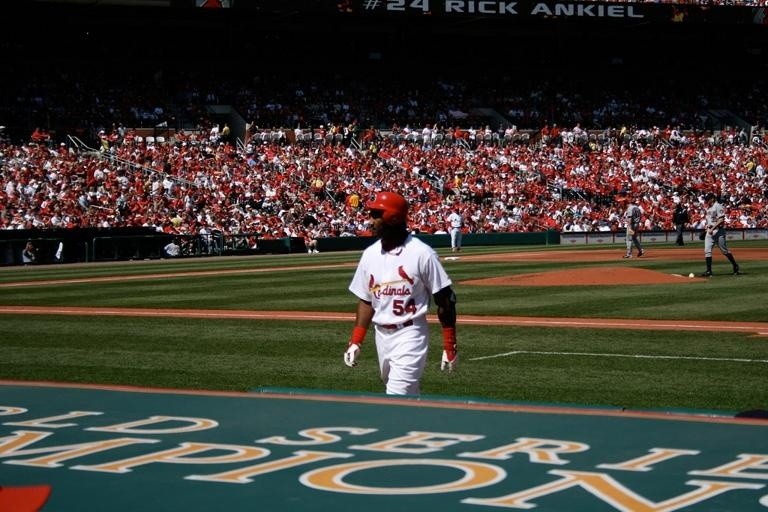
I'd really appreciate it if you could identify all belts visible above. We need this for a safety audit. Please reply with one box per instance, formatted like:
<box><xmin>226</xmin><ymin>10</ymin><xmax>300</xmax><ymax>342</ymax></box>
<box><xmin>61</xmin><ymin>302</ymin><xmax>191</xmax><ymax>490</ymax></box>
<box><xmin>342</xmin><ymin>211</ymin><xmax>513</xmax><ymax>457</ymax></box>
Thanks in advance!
<box><xmin>383</xmin><ymin>320</ymin><xmax>414</xmax><ymax>330</ymax></box>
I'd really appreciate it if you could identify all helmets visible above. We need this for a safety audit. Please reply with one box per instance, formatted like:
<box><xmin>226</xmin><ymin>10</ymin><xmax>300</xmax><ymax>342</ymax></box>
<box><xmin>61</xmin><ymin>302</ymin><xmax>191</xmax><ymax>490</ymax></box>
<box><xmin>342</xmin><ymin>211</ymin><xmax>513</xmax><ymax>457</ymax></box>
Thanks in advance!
<box><xmin>365</xmin><ymin>191</ymin><xmax>409</xmax><ymax>225</ymax></box>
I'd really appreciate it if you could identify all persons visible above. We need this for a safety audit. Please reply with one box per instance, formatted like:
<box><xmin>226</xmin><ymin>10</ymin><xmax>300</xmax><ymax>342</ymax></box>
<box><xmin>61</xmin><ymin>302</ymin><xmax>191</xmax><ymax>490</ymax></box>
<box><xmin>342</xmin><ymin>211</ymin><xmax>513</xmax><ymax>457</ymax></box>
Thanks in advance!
<box><xmin>0</xmin><ymin>81</ymin><xmax>768</xmax><ymax>276</ymax></box>
<box><xmin>343</xmin><ymin>191</ymin><xmax>457</xmax><ymax>395</ymax></box>
<box><xmin>703</xmin><ymin>192</ymin><xmax>739</xmax><ymax>274</ymax></box>
<box><xmin>672</xmin><ymin>202</ymin><xmax>689</xmax><ymax>246</ymax></box>
<box><xmin>304</xmin><ymin>222</ymin><xmax>321</xmax><ymax>254</ymax></box>
<box><xmin>446</xmin><ymin>209</ymin><xmax>465</xmax><ymax>252</ymax></box>
<box><xmin>622</xmin><ymin>199</ymin><xmax>645</xmax><ymax>258</ymax></box>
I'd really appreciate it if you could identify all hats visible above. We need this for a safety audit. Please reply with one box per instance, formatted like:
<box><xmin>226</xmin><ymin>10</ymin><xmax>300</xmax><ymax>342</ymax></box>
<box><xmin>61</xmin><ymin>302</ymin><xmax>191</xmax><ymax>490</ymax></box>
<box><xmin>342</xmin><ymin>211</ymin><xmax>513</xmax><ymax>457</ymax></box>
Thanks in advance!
<box><xmin>703</xmin><ymin>193</ymin><xmax>714</xmax><ymax>199</ymax></box>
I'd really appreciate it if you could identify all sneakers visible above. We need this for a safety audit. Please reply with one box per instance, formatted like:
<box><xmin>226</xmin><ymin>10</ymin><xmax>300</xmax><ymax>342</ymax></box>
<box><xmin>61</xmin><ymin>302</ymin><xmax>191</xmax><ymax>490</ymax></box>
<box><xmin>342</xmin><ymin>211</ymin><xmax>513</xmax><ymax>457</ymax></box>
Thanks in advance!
<box><xmin>701</xmin><ymin>271</ymin><xmax>712</xmax><ymax>276</ymax></box>
<box><xmin>733</xmin><ymin>264</ymin><xmax>739</xmax><ymax>272</ymax></box>
<box><xmin>637</xmin><ymin>249</ymin><xmax>644</xmax><ymax>257</ymax></box>
<box><xmin>623</xmin><ymin>255</ymin><xmax>632</xmax><ymax>258</ymax></box>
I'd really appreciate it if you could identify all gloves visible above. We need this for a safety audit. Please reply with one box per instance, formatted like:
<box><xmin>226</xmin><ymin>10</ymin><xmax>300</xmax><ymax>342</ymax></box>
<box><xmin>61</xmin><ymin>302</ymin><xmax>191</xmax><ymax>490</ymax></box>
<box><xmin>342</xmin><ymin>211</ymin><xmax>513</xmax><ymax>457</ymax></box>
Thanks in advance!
<box><xmin>441</xmin><ymin>350</ymin><xmax>457</xmax><ymax>374</ymax></box>
<box><xmin>343</xmin><ymin>343</ymin><xmax>362</xmax><ymax>368</ymax></box>
